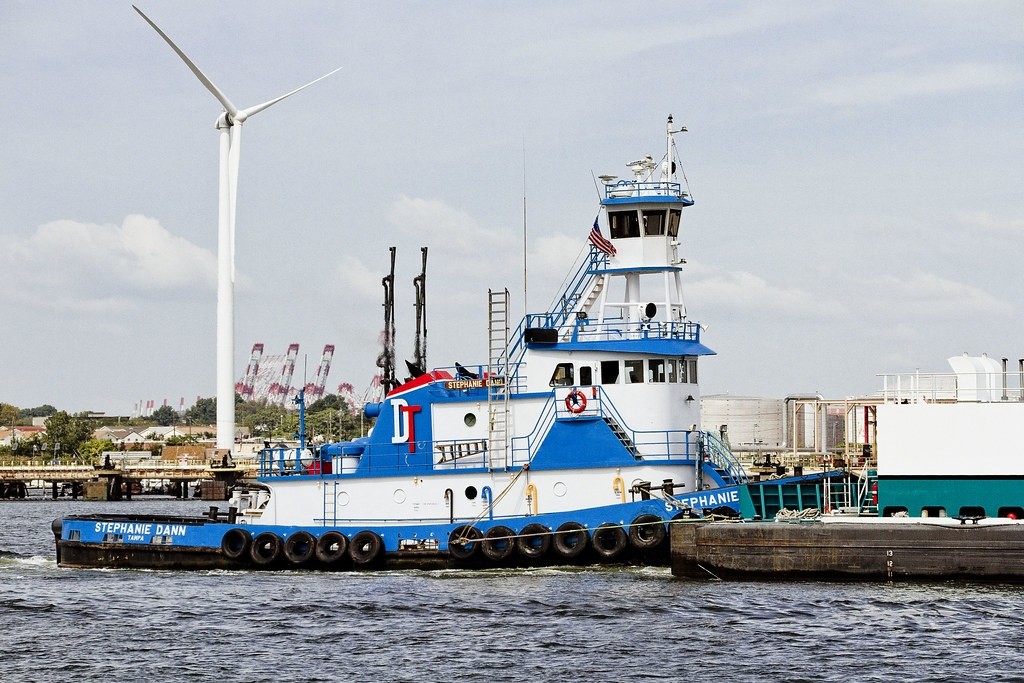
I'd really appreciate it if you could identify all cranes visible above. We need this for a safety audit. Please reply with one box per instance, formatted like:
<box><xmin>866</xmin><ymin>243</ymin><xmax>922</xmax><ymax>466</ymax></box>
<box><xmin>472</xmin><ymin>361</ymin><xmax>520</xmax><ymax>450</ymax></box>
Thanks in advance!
<box><xmin>132</xmin><ymin>397</ymin><xmax>186</xmax><ymax>419</ymax></box>
<box><xmin>238</xmin><ymin>331</ymin><xmax>392</xmax><ymax>416</ymax></box>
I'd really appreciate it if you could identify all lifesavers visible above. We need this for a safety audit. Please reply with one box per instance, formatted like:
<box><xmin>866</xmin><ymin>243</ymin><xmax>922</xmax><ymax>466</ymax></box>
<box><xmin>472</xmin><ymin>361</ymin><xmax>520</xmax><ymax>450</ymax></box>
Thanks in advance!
<box><xmin>448</xmin><ymin>506</ymin><xmax>739</xmax><ymax>559</ymax></box>
<box><xmin>565</xmin><ymin>391</ymin><xmax>587</xmax><ymax>413</ymax></box>
<box><xmin>221</xmin><ymin>528</ymin><xmax>385</xmax><ymax>565</ymax></box>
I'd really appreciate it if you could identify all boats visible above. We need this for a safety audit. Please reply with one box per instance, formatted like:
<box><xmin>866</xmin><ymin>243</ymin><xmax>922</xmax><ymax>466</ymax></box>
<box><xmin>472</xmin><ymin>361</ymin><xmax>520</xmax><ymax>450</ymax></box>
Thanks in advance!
<box><xmin>50</xmin><ymin>113</ymin><xmax>760</xmax><ymax>569</ymax></box>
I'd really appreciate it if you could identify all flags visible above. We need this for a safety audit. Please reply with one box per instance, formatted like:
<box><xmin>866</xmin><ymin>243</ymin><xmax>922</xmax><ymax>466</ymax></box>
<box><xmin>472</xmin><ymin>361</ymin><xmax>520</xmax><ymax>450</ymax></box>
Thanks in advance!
<box><xmin>588</xmin><ymin>215</ymin><xmax>617</xmax><ymax>257</ymax></box>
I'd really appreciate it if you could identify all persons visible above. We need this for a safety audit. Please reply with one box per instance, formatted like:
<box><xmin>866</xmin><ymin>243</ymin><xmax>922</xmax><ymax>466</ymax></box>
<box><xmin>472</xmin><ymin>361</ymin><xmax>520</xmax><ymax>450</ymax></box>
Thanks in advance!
<box><xmin>222</xmin><ymin>454</ymin><xmax>228</xmax><ymax>468</ymax></box>
<box><xmin>628</xmin><ymin>218</ymin><xmax>648</xmax><ymax>236</ymax></box>
<box><xmin>103</xmin><ymin>455</ymin><xmax>112</xmax><ymax>470</ymax></box>
